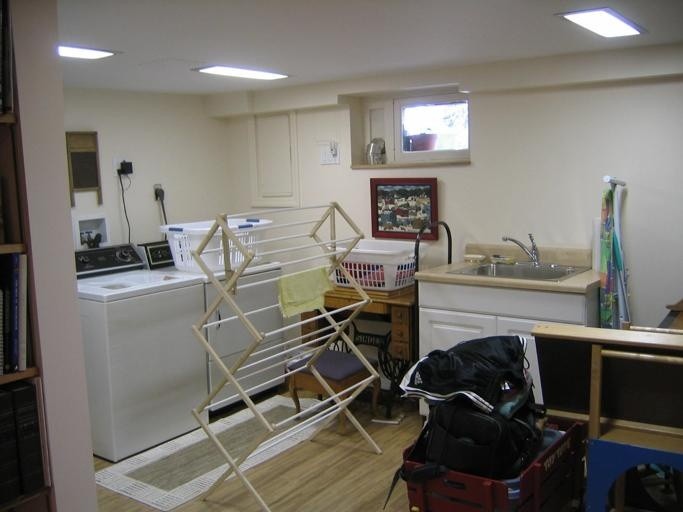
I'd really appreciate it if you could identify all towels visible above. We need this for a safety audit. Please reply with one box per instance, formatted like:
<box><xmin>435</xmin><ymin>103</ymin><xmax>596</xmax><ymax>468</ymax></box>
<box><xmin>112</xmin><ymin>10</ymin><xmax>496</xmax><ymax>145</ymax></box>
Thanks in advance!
<box><xmin>276</xmin><ymin>265</ymin><xmax>334</xmax><ymax>319</ymax></box>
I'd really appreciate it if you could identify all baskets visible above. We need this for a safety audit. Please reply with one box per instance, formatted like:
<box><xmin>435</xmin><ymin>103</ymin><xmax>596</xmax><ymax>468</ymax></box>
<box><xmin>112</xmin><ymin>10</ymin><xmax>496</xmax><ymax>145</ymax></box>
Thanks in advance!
<box><xmin>325</xmin><ymin>239</ymin><xmax>415</xmax><ymax>292</ymax></box>
<box><xmin>160</xmin><ymin>219</ymin><xmax>272</xmax><ymax>274</ymax></box>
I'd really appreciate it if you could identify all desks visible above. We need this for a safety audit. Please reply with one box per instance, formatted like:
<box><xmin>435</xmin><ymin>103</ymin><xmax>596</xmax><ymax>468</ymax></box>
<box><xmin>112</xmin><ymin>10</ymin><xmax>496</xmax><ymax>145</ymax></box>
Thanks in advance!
<box><xmin>301</xmin><ymin>290</ymin><xmax>416</xmax><ymax>405</ymax></box>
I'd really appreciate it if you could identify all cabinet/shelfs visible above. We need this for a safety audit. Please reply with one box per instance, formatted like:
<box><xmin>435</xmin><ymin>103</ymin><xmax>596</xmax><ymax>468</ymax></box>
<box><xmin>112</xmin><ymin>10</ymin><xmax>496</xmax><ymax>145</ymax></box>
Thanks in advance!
<box><xmin>417</xmin><ymin>279</ymin><xmax>601</xmax><ymax>429</ymax></box>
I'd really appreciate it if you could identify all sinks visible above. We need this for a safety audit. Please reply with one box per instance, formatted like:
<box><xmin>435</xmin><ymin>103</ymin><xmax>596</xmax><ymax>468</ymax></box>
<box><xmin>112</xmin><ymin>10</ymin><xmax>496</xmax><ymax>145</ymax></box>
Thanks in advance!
<box><xmin>446</xmin><ymin>258</ymin><xmax>583</xmax><ymax>281</ymax></box>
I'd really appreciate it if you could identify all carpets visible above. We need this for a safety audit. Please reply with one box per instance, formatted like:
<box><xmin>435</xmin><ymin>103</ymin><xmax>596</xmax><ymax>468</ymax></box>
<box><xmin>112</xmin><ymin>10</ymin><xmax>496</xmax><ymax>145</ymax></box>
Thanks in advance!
<box><xmin>96</xmin><ymin>394</ymin><xmax>339</xmax><ymax>511</ymax></box>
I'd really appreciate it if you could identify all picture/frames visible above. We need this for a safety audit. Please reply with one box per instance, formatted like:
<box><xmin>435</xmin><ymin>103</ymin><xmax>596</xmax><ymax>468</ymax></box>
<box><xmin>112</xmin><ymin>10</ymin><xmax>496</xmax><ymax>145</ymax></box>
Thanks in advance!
<box><xmin>370</xmin><ymin>177</ymin><xmax>439</xmax><ymax>241</ymax></box>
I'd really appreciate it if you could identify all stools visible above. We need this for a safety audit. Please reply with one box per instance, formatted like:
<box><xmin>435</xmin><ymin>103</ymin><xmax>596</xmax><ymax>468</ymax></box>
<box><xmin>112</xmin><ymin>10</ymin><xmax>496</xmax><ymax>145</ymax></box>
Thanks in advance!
<box><xmin>285</xmin><ymin>346</ymin><xmax>388</xmax><ymax>437</ymax></box>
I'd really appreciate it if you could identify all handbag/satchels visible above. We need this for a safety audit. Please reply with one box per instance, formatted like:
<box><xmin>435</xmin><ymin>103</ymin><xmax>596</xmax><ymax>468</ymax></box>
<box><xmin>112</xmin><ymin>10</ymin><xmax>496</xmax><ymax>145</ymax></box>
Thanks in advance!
<box><xmin>406</xmin><ymin>336</ymin><xmax>546</xmax><ymax>479</ymax></box>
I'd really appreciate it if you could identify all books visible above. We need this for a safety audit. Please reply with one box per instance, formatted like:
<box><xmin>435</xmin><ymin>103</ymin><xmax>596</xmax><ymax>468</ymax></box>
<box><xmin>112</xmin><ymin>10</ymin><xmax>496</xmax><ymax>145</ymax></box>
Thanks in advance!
<box><xmin>10</xmin><ymin>251</ymin><xmax>31</xmax><ymax>373</ymax></box>
<box><xmin>0</xmin><ymin>386</ymin><xmax>46</xmax><ymax>502</ymax></box>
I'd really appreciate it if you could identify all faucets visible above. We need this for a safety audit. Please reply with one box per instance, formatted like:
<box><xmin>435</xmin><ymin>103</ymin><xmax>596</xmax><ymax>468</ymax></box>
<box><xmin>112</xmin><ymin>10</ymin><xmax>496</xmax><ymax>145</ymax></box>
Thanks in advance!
<box><xmin>500</xmin><ymin>234</ymin><xmax>539</xmax><ymax>265</ymax></box>
